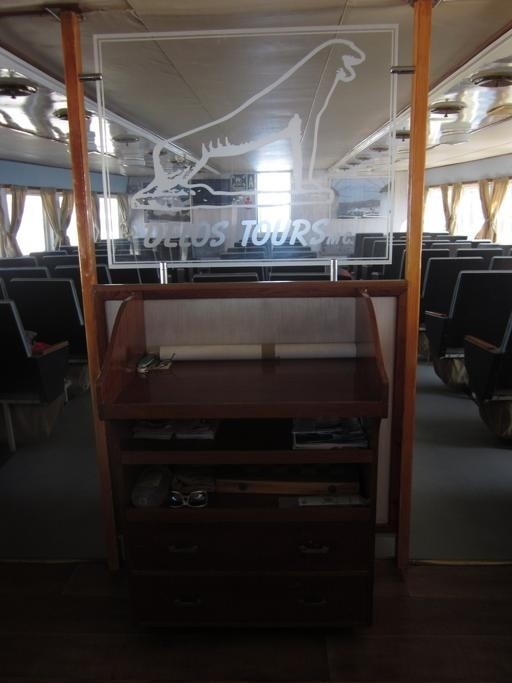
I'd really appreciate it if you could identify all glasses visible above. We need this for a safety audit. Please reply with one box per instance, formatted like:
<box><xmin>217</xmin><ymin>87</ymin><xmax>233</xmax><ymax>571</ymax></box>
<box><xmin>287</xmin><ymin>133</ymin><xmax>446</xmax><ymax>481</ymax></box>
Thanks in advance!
<box><xmin>165</xmin><ymin>491</ymin><xmax>208</xmax><ymax>508</ymax></box>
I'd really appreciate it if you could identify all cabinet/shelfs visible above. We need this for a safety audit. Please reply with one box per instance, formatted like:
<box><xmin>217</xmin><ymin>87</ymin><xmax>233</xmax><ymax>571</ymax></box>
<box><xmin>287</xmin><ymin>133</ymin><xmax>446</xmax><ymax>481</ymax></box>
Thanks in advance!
<box><xmin>95</xmin><ymin>287</ymin><xmax>390</xmax><ymax>630</ymax></box>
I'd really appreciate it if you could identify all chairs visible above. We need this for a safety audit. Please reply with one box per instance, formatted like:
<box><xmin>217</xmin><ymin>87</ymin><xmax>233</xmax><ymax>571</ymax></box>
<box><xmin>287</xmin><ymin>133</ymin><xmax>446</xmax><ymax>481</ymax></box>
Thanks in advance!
<box><xmin>0</xmin><ymin>238</ymin><xmax>331</xmax><ymax>448</ymax></box>
<box><xmin>337</xmin><ymin>229</ymin><xmax>511</xmax><ymax>442</ymax></box>
